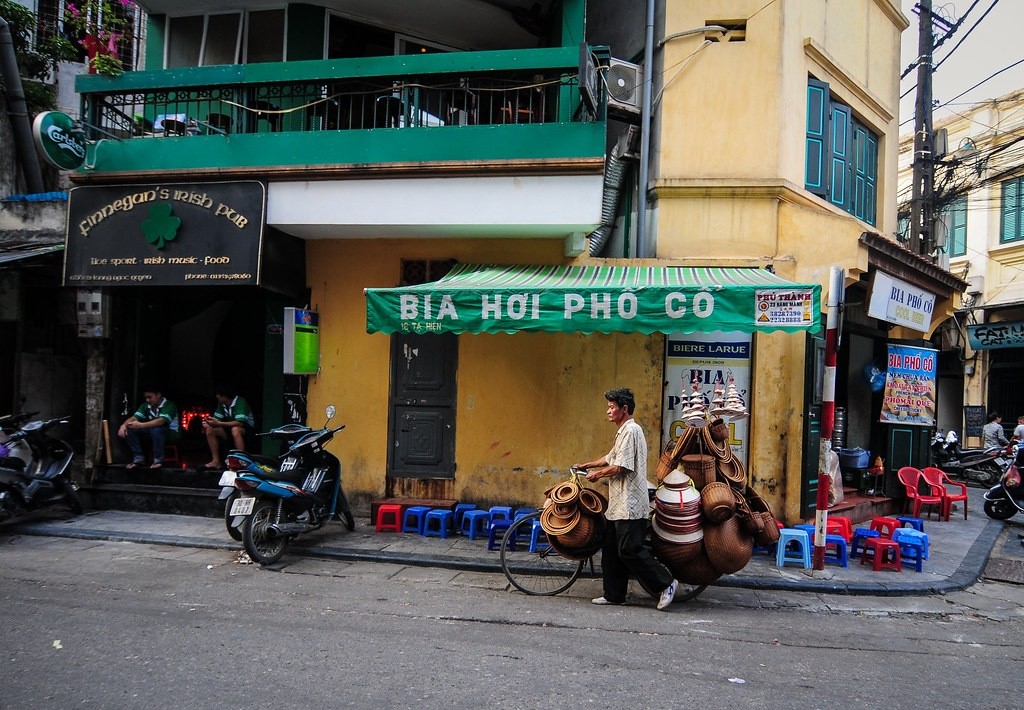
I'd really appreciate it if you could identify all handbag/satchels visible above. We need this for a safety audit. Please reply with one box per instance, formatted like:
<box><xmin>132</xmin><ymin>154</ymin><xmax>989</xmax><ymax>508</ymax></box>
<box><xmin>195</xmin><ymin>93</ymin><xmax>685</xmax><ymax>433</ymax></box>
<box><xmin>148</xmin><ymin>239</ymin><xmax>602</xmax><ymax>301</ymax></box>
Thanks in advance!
<box><xmin>1006</xmin><ymin>465</ymin><xmax>1021</xmax><ymax>487</ymax></box>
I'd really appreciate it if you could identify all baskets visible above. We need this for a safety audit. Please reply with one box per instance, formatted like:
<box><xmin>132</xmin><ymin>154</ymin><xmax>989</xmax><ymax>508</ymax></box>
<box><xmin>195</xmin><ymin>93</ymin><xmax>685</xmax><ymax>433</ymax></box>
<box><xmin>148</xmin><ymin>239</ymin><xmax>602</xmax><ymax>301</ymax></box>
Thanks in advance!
<box><xmin>540</xmin><ymin>423</ymin><xmax>779</xmax><ymax>585</ymax></box>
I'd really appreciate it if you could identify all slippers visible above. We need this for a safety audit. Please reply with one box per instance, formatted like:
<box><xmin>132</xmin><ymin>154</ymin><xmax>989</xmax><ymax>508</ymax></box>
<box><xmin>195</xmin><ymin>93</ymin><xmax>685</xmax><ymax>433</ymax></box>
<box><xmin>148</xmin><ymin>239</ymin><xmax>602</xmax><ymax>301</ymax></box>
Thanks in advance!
<box><xmin>198</xmin><ymin>464</ymin><xmax>216</xmax><ymax>471</ymax></box>
<box><xmin>126</xmin><ymin>462</ymin><xmax>142</xmax><ymax>469</ymax></box>
<box><xmin>149</xmin><ymin>462</ymin><xmax>162</xmax><ymax>469</ymax></box>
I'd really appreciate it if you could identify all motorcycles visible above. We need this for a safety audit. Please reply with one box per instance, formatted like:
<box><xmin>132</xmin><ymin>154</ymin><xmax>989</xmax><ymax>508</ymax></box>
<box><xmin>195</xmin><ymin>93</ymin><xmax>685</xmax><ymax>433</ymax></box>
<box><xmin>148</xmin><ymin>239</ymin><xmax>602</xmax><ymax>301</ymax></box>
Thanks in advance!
<box><xmin>225</xmin><ymin>403</ymin><xmax>356</xmax><ymax>564</ymax></box>
<box><xmin>218</xmin><ymin>423</ymin><xmax>319</xmax><ymax>542</ymax></box>
<box><xmin>0</xmin><ymin>410</ymin><xmax>83</xmax><ymax>522</ymax></box>
<box><xmin>929</xmin><ymin>427</ymin><xmax>1008</xmax><ymax>489</ymax></box>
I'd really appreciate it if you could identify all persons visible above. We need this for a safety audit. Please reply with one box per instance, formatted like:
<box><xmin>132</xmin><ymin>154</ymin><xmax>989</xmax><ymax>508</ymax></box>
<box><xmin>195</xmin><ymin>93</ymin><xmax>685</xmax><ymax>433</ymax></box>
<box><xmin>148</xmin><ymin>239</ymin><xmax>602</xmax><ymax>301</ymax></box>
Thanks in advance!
<box><xmin>199</xmin><ymin>382</ymin><xmax>259</xmax><ymax>471</ymax></box>
<box><xmin>572</xmin><ymin>387</ymin><xmax>678</xmax><ymax>609</ymax></box>
<box><xmin>982</xmin><ymin>411</ymin><xmax>1010</xmax><ymax>449</ymax></box>
<box><xmin>1014</xmin><ymin>416</ymin><xmax>1024</xmax><ymax>440</ymax></box>
<box><xmin>117</xmin><ymin>384</ymin><xmax>180</xmax><ymax>469</ymax></box>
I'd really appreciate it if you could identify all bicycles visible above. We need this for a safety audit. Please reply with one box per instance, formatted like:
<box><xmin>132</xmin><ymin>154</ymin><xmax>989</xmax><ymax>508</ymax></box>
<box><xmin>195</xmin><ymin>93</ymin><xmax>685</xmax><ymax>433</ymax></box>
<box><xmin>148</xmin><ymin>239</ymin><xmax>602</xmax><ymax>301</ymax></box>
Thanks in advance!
<box><xmin>500</xmin><ymin>466</ymin><xmax>709</xmax><ymax>603</ymax></box>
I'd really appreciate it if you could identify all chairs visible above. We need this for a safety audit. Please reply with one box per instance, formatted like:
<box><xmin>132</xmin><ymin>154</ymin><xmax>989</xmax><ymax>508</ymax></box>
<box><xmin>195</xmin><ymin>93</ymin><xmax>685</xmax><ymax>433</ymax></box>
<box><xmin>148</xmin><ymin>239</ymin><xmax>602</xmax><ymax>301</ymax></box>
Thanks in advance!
<box><xmin>898</xmin><ymin>467</ymin><xmax>945</xmax><ymax>521</ymax></box>
<box><xmin>928</xmin><ymin>464</ymin><xmax>968</xmax><ymax>520</ymax></box>
<box><xmin>161</xmin><ymin>93</ymin><xmax>537</xmax><ymax>133</ymax></box>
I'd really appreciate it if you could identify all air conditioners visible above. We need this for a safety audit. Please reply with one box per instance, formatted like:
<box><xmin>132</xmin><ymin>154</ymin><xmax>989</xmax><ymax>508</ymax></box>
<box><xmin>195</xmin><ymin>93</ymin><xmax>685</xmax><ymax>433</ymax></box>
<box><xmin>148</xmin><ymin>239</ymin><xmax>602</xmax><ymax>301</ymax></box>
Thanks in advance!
<box><xmin>590</xmin><ymin>53</ymin><xmax>653</xmax><ymax>117</ymax></box>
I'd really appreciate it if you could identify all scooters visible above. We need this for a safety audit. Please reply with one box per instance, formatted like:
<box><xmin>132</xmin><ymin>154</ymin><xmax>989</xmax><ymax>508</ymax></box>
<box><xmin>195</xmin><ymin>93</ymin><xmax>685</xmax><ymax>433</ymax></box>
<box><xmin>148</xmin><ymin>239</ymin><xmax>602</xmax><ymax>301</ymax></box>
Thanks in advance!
<box><xmin>983</xmin><ymin>438</ymin><xmax>1024</xmax><ymax>521</ymax></box>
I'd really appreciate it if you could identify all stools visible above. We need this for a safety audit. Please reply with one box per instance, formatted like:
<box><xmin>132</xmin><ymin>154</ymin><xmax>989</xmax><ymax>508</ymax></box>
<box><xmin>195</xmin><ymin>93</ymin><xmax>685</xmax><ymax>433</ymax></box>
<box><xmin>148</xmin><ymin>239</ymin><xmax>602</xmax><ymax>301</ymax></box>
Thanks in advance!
<box><xmin>369</xmin><ymin>502</ymin><xmax>932</xmax><ymax>572</ymax></box>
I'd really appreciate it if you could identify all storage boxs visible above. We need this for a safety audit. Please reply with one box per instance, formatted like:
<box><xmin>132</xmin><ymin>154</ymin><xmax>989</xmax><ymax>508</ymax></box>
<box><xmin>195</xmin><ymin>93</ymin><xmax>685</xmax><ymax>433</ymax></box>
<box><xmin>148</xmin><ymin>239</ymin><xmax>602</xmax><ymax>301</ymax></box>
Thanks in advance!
<box><xmin>842</xmin><ymin>448</ymin><xmax>874</xmax><ymax>488</ymax></box>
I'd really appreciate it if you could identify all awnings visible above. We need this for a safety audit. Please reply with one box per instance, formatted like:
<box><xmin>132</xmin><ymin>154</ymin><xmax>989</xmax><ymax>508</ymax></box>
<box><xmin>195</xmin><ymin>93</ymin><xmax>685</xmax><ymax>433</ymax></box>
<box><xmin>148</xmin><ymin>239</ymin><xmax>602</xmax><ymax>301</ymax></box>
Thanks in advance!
<box><xmin>363</xmin><ymin>263</ymin><xmax>822</xmax><ymax>336</ymax></box>
<box><xmin>953</xmin><ymin>297</ymin><xmax>1024</xmax><ymax>312</ymax></box>
<box><xmin>0</xmin><ymin>244</ymin><xmax>65</xmax><ymax>263</ymax></box>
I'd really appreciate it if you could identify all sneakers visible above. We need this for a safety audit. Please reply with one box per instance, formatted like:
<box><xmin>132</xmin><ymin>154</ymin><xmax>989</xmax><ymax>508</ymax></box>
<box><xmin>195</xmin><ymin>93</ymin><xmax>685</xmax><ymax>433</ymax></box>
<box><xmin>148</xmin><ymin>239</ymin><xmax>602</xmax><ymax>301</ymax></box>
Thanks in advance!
<box><xmin>657</xmin><ymin>579</ymin><xmax>679</xmax><ymax>609</ymax></box>
<box><xmin>592</xmin><ymin>596</ymin><xmax>626</xmax><ymax>605</ymax></box>
